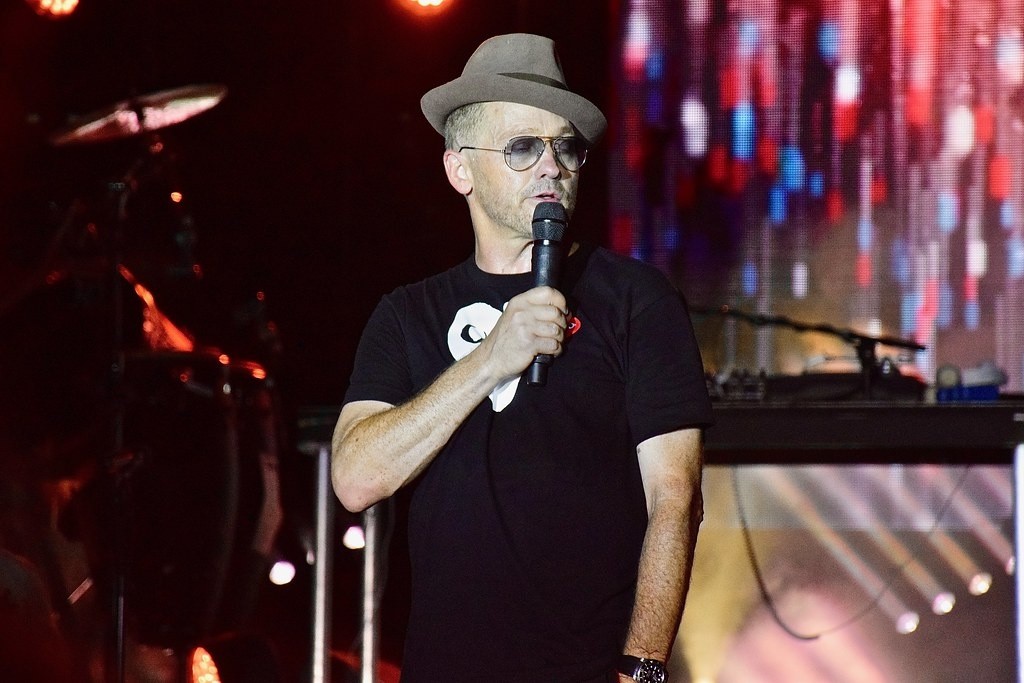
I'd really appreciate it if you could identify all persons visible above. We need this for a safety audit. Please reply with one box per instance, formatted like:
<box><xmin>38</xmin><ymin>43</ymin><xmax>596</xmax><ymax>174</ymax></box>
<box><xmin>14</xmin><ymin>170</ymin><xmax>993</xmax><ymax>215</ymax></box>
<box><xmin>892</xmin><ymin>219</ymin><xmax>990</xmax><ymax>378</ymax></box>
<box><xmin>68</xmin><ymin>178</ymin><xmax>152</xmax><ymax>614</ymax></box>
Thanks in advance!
<box><xmin>329</xmin><ymin>33</ymin><xmax>719</xmax><ymax>683</ymax></box>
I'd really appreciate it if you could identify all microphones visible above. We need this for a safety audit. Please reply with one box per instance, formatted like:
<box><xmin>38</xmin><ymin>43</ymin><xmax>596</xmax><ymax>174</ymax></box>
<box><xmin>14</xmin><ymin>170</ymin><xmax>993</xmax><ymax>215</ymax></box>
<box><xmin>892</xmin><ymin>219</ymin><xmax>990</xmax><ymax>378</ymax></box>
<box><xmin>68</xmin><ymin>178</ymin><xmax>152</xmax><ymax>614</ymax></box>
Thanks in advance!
<box><xmin>526</xmin><ymin>202</ymin><xmax>570</xmax><ymax>387</ymax></box>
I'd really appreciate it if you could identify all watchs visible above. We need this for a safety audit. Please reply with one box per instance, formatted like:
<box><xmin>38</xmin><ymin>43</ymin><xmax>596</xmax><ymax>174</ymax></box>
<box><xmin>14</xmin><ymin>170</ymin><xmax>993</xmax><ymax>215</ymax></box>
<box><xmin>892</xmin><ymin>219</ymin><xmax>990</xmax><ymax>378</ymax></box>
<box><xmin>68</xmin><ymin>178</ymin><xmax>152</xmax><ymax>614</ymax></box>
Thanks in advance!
<box><xmin>614</xmin><ymin>655</ymin><xmax>668</xmax><ymax>683</ymax></box>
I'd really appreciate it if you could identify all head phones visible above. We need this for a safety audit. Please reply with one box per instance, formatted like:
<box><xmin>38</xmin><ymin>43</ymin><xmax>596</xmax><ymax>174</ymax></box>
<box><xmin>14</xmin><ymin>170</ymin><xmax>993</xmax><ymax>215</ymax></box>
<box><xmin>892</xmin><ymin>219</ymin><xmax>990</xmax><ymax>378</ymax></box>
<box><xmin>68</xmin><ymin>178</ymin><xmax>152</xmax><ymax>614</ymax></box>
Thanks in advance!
<box><xmin>936</xmin><ymin>360</ymin><xmax>1007</xmax><ymax>387</ymax></box>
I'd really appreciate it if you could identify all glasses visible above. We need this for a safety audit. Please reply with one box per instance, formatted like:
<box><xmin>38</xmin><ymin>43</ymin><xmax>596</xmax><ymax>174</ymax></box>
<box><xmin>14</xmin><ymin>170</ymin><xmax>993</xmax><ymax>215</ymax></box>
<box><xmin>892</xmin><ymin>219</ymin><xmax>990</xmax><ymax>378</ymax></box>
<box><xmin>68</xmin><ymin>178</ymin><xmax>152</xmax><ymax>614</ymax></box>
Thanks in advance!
<box><xmin>459</xmin><ymin>135</ymin><xmax>588</xmax><ymax>172</ymax></box>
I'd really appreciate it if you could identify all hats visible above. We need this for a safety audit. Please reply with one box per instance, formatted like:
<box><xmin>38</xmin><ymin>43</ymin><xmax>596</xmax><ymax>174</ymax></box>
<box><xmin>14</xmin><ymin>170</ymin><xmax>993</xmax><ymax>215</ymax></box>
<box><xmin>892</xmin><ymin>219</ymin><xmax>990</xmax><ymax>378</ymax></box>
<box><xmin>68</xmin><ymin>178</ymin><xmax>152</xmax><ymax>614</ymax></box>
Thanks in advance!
<box><xmin>419</xmin><ymin>33</ymin><xmax>607</xmax><ymax>152</ymax></box>
